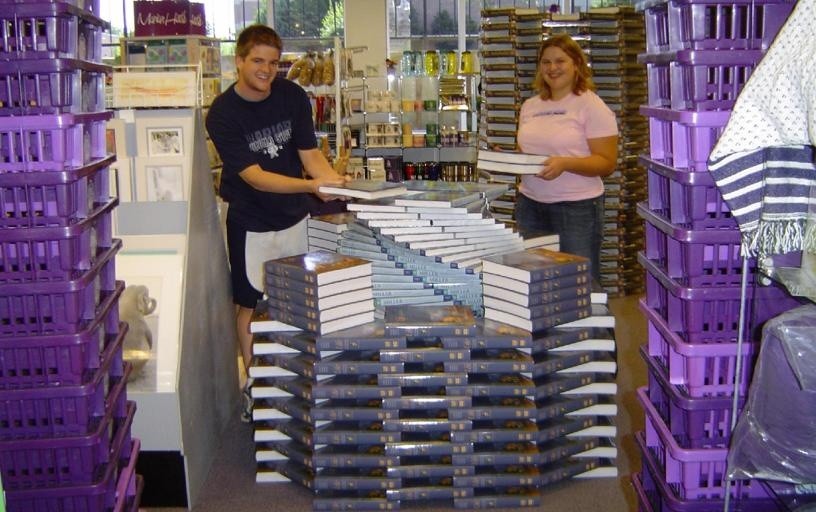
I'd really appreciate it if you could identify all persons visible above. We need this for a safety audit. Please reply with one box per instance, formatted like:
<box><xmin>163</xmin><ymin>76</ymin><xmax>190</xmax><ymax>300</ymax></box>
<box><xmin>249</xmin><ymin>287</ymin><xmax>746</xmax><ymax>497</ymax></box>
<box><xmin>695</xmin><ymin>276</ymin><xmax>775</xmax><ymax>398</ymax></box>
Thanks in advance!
<box><xmin>205</xmin><ymin>25</ymin><xmax>351</xmax><ymax>422</ymax></box>
<box><xmin>516</xmin><ymin>34</ymin><xmax>620</xmax><ymax>287</ymax></box>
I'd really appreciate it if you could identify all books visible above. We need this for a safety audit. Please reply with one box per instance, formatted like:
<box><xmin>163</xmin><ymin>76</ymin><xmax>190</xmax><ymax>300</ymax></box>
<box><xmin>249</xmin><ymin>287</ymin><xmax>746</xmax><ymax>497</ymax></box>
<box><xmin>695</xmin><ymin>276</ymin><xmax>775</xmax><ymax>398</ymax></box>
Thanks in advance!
<box><xmin>473</xmin><ymin>5</ymin><xmax>649</xmax><ymax>181</ymax></box>
<box><xmin>475</xmin><ymin>149</ymin><xmax>552</xmax><ymax>176</ymax></box>
<box><xmin>245</xmin><ymin>179</ymin><xmax>647</xmax><ymax>510</ymax></box>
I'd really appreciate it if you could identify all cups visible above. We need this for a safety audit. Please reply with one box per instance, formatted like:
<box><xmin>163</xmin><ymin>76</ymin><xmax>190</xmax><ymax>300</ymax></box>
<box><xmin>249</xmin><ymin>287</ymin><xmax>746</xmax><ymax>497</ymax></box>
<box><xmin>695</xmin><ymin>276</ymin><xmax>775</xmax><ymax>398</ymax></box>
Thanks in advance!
<box><xmin>367</xmin><ymin>89</ymin><xmax>400</xmax><ymax>113</ymax></box>
<box><xmin>406</xmin><ymin>175</ymin><xmax>437</xmax><ymax>181</ymax></box>
<box><xmin>401</xmin><ymin>100</ymin><xmax>438</xmax><ymax>112</ymax></box>
<box><xmin>403</xmin><ymin>123</ymin><xmax>436</xmax><ymax>147</ymax></box>
<box><xmin>368</xmin><ymin>123</ymin><xmax>400</xmax><ymax>145</ymax></box>
<box><xmin>404</xmin><ymin>161</ymin><xmax>437</xmax><ymax>181</ymax></box>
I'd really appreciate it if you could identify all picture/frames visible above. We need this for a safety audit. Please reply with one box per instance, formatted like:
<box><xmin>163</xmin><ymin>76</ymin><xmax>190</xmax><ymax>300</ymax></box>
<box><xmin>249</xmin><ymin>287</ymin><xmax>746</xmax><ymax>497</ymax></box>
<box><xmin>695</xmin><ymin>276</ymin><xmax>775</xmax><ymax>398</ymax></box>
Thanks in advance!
<box><xmin>135</xmin><ymin>156</ymin><xmax>190</xmax><ymax>204</ymax></box>
<box><xmin>135</xmin><ymin>118</ymin><xmax>194</xmax><ymax>155</ymax></box>
<box><xmin>110</xmin><ymin>159</ymin><xmax>131</xmax><ymax>202</ymax></box>
<box><xmin>106</xmin><ymin>121</ymin><xmax>125</xmax><ymax>160</ymax></box>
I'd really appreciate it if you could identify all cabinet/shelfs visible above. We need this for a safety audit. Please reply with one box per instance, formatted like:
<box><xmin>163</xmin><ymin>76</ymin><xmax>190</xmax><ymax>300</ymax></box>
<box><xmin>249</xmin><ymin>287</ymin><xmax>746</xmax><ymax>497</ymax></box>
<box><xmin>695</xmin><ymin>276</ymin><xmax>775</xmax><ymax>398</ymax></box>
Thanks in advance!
<box><xmin>108</xmin><ymin>67</ymin><xmax>242</xmax><ymax>511</ymax></box>
<box><xmin>119</xmin><ymin>37</ymin><xmax>224</xmax><ymax>205</ymax></box>
<box><xmin>217</xmin><ymin>37</ymin><xmax>480</xmax><ymax>183</ymax></box>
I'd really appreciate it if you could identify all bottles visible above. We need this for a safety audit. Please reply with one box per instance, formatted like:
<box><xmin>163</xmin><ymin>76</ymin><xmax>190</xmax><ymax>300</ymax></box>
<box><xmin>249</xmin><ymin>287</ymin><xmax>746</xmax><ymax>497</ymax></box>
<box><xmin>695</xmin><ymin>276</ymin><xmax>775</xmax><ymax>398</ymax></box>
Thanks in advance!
<box><xmin>442</xmin><ymin>175</ymin><xmax>478</xmax><ymax>184</ymax></box>
<box><xmin>439</xmin><ymin>161</ymin><xmax>475</xmax><ymax>175</ymax></box>
<box><xmin>439</xmin><ymin>125</ymin><xmax>474</xmax><ymax>147</ymax></box>
<box><xmin>439</xmin><ymin>96</ymin><xmax>466</xmax><ymax>111</ymax></box>
<box><xmin>401</xmin><ymin>50</ymin><xmax>475</xmax><ymax>76</ymax></box>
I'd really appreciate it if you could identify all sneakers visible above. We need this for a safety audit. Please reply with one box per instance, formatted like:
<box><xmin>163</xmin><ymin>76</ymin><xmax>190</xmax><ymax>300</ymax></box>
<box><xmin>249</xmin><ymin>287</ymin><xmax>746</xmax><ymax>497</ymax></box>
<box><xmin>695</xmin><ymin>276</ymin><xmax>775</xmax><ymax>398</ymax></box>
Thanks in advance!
<box><xmin>240</xmin><ymin>385</ymin><xmax>253</xmax><ymax>423</ymax></box>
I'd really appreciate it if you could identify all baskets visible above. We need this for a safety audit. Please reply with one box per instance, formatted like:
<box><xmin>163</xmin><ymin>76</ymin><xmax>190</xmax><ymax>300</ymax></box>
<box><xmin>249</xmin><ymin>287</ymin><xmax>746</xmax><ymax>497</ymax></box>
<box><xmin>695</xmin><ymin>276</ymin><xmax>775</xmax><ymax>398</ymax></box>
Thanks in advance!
<box><xmin>1</xmin><ymin>1</ymin><xmax>144</xmax><ymax>512</ymax></box>
<box><xmin>631</xmin><ymin>1</ymin><xmax>812</xmax><ymax>512</ymax></box>
<box><xmin>631</xmin><ymin>0</ymin><xmax>812</xmax><ymax>512</ymax></box>
<box><xmin>1</xmin><ymin>0</ymin><xmax>144</xmax><ymax>512</ymax></box>
